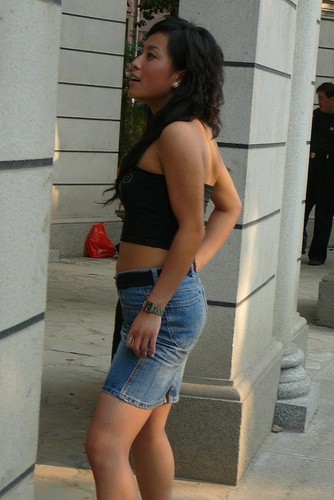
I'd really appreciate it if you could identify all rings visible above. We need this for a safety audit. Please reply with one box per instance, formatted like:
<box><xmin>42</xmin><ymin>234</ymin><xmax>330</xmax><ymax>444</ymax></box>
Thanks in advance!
<box><xmin>126</xmin><ymin>334</ymin><xmax>134</xmax><ymax>346</ymax></box>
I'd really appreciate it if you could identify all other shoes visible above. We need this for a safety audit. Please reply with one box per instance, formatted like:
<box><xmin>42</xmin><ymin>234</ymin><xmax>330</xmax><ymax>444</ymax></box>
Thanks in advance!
<box><xmin>302</xmin><ymin>248</ymin><xmax>307</xmax><ymax>254</ymax></box>
<box><xmin>308</xmin><ymin>260</ymin><xmax>322</xmax><ymax>265</ymax></box>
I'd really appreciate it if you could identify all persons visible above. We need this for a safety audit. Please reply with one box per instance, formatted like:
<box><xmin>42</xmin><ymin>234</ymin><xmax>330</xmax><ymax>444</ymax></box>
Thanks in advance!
<box><xmin>302</xmin><ymin>83</ymin><xmax>334</xmax><ymax>266</ymax></box>
<box><xmin>85</xmin><ymin>18</ymin><xmax>243</xmax><ymax>500</ymax></box>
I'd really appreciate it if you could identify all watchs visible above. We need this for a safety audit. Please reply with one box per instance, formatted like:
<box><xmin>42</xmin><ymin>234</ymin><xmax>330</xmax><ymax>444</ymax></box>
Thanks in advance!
<box><xmin>141</xmin><ymin>300</ymin><xmax>165</xmax><ymax>316</ymax></box>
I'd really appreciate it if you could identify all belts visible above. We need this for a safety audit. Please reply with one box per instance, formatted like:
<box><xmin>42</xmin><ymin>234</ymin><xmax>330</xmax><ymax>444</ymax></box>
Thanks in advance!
<box><xmin>116</xmin><ymin>263</ymin><xmax>196</xmax><ymax>290</ymax></box>
